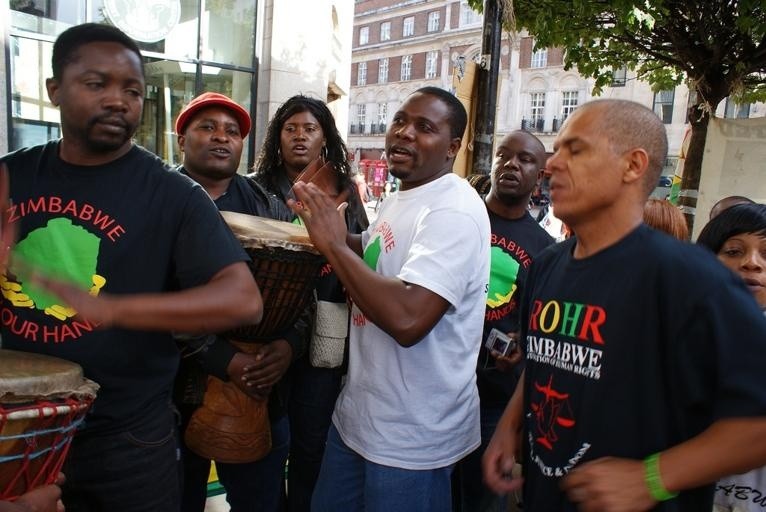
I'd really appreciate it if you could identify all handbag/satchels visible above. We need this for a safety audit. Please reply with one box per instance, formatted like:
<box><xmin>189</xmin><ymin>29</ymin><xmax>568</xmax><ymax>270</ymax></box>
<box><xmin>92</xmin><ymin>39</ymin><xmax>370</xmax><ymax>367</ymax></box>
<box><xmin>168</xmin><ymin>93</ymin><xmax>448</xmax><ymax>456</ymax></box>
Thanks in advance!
<box><xmin>310</xmin><ymin>298</ymin><xmax>349</xmax><ymax>370</ymax></box>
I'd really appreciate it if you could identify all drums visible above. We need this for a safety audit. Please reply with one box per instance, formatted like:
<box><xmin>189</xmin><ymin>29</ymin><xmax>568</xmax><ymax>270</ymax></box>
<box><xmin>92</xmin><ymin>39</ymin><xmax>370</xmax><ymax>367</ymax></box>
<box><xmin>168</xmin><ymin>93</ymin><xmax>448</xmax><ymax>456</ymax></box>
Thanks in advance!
<box><xmin>0</xmin><ymin>349</ymin><xmax>100</xmax><ymax>501</ymax></box>
<box><xmin>183</xmin><ymin>212</ymin><xmax>331</xmax><ymax>463</ymax></box>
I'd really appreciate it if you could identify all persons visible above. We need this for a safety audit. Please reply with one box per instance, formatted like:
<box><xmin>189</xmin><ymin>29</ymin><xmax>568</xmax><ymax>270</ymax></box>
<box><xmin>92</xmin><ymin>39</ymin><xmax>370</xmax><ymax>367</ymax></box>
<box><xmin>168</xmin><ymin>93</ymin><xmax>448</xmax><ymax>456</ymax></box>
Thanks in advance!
<box><xmin>450</xmin><ymin>128</ymin><xmax>544</xmax><ymax>511</ymax></box>
<box><xmin>0</xmin><ymin>21</ymin><xmax>267</xmax><ymax>511</ymax></box>
<box><xmin>284</xmin><ymin>86</ymin><xmax>493</xmax><ymax>512</ymax></box>
<box><xmin>479</xmin><ymin>94</ymin><xmax>766</xmax><ymax>511</ymax></box>
<box><xmin>163</xmin><ymin>90</ymin><xmax>318</xmax><ymax>511</ymax></box>
<box><xmin>244</xmin><ymin>95</ymin><xmax>370</xmax><ymax>512</ymax></box>
<box><xmin>694</xmin><ymin>202</ymin><xmax>766</xmax><ymax>512</ymax></box>
<box><xmin>524</xmin><ymin>172</ymin><xmax>766</xmax><ymax>251</ymax></box>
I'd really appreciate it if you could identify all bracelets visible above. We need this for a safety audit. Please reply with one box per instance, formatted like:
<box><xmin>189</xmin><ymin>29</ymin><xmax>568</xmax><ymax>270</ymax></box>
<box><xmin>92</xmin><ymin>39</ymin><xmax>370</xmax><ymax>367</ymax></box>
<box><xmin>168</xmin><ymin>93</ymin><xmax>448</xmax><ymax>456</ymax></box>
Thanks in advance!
<box><xmin>642</xmin><ymin>452</ymin><xmax>678</xmax><ymax>503</ymax></box>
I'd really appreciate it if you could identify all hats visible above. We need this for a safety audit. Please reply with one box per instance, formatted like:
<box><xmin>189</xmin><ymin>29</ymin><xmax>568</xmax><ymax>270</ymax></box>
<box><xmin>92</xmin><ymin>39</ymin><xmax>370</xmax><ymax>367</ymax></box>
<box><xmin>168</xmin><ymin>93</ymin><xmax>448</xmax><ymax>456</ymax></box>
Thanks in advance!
<box><xmin>175</xmin><ymin>92</ymin><xmax>250</xmax><ymax>139</ymax></box>
